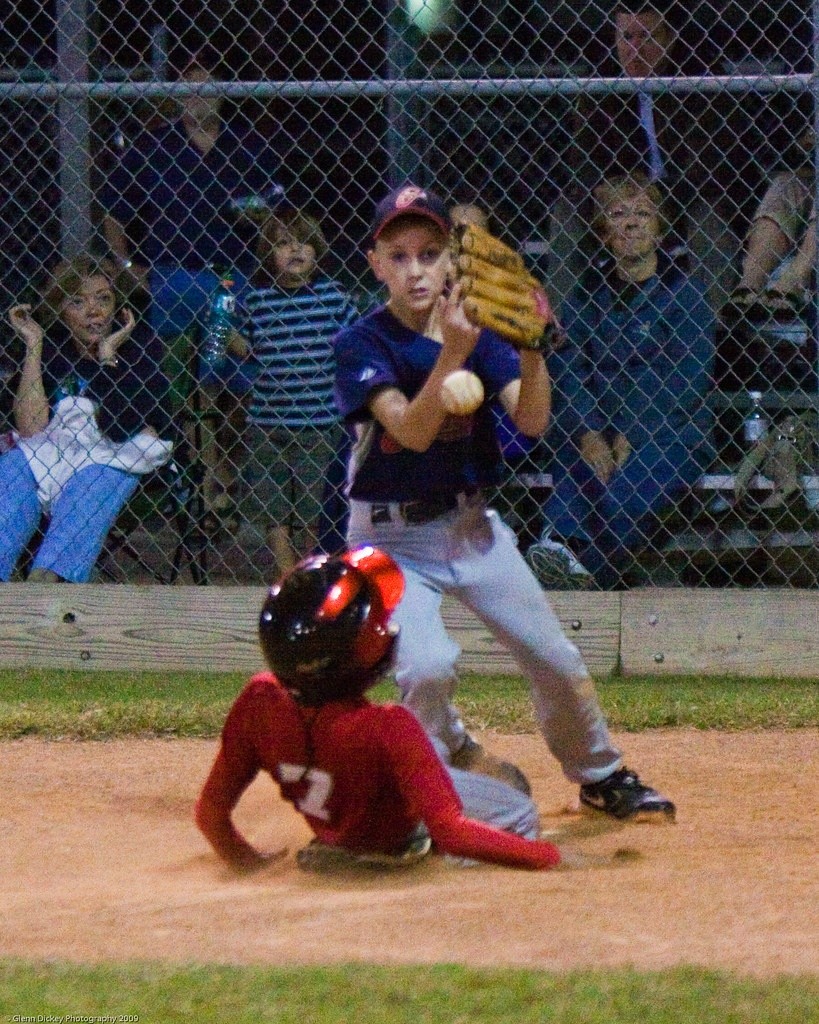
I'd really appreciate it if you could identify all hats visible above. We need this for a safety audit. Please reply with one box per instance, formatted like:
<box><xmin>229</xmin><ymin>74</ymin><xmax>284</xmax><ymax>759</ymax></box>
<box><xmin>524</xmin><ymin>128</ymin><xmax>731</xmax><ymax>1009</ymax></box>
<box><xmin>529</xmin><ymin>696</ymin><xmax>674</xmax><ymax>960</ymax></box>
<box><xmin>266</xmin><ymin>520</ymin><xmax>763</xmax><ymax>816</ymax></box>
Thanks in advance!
<box><xmin>373</xmin><ymin>187</ymin><xmax>453</xmax><ymax>244</ymax></box>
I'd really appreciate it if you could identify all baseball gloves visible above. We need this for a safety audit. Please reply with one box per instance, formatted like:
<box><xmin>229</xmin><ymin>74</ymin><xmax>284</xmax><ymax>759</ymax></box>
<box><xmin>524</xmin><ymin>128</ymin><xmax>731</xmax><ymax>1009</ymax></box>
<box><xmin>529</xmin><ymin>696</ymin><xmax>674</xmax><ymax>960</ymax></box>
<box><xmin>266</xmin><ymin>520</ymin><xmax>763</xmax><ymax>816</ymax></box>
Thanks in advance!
<box><xmin>458</xmin><ymin>221</ymin><xmax>561</xmax><ymax>354</ymax></box>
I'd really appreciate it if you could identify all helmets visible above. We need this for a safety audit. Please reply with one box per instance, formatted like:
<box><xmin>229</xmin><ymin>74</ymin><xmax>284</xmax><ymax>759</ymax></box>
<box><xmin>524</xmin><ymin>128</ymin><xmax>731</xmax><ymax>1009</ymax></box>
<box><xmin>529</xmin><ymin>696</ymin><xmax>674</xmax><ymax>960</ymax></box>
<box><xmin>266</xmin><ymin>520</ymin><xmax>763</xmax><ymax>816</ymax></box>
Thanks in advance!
<box><xmin>257</xmin><ymin>546</ymin><xmax>406</xmax><ymax>700</ymax></box>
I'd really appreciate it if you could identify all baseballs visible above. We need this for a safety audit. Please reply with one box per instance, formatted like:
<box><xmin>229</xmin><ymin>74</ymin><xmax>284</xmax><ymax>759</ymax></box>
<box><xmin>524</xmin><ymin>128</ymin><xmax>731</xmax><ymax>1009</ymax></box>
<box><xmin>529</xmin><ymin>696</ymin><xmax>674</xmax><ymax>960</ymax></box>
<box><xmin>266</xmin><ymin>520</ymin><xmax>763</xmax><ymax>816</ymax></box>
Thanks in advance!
<box><xmin>438</xmin><ymin>369</ymin><xmax>485</xmax><ymax>418</ymax></box>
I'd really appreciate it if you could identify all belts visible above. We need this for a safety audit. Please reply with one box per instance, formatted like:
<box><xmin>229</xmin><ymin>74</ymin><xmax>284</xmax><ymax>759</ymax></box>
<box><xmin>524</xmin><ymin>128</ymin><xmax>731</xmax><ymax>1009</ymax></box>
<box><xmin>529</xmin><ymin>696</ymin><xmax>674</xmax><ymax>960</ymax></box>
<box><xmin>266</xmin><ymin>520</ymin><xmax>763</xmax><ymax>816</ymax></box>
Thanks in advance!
<box><xmin>373</xmin><ymin>492</ymin><xmax>461</xmax><ymax>528</ymax></box>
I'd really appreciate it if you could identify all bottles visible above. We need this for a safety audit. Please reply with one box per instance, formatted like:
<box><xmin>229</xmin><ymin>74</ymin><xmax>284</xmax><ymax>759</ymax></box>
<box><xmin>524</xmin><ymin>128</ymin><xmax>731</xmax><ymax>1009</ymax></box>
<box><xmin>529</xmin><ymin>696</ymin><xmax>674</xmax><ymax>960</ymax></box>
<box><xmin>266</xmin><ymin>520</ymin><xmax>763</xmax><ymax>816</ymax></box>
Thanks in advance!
<box><xmin>201</xmin><ymin>279</ymin><xmax>235</xmax><ymax>370</ymax></box>
<box><xmin>229</xmin><ymin>183</ymin><xmax>284</xmax><ymax>211</ymax></box>
<box><xmin>113</xmin><ymin>123</ymin><xmax>134</xmax><ymax>170</ymax></box>
<box><xmin>743</xmin><ymin>391</ymin><xmax>769</xmax><ymax>448</ymax></box>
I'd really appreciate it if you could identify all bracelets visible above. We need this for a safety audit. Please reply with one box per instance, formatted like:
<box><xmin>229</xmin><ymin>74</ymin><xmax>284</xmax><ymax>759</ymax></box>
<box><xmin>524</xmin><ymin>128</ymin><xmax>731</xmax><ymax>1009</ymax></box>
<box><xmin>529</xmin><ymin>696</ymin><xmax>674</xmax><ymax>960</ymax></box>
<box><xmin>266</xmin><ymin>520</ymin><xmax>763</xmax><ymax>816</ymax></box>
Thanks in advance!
<box><xmin>103</xmin><ymin>358</ymin><xmax>119</xmax><ymax>366</ymax></box>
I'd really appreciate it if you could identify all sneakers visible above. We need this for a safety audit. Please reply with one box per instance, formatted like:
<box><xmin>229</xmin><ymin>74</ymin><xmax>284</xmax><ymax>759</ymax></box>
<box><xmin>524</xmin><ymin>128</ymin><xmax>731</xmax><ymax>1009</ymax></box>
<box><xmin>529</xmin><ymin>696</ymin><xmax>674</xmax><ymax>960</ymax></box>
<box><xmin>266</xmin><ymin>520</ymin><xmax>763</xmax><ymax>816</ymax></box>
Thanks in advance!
<box><xmin>297</xmin><ymin>836</ymin><xmax>434</xmax><ymax>875</ymax></box>
<box><xmin>452</xmin><ymin>739</ymin><xmax>533</xmax><ymax>800</ymax></box>
<box><xmin>524</xmin><ymin>540</ymin><xmax>591</xmax><ymax>588</ymax></box>
<box><xmin>578</xmin><ymin>771</ymin><xmax>677</xmax><ymax>826</ymax></box>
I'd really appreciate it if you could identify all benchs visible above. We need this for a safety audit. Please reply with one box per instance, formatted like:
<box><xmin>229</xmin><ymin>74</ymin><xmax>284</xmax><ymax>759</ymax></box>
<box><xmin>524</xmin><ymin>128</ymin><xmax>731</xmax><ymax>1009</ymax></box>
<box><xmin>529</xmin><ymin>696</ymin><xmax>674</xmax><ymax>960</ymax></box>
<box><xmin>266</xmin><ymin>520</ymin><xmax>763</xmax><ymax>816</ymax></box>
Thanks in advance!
<box><xmin>509</xmin><ymin>241</ymin><xmax>819</xmax><ymax>559</ymax></box>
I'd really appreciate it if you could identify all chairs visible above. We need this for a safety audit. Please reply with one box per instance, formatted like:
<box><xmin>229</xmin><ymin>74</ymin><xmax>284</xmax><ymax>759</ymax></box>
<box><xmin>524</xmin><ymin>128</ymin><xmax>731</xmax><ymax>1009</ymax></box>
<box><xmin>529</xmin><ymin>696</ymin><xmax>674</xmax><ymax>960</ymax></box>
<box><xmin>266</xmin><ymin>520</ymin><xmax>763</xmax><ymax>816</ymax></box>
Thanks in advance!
<box><xmin>57</xmin><ymin>271</ymin><xmax>234</xmax><ymax>584</ymax></box>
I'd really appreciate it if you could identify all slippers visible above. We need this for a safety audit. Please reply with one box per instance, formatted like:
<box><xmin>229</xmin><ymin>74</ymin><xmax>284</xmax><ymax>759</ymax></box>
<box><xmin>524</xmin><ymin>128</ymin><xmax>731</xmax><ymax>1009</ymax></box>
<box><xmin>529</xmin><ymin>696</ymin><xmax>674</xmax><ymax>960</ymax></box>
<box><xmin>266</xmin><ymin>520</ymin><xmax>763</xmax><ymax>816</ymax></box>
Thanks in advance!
<box><xmin>722</xmin><ymin>285</ymin><xmax>800</xmax><ymax>319</ymax></box>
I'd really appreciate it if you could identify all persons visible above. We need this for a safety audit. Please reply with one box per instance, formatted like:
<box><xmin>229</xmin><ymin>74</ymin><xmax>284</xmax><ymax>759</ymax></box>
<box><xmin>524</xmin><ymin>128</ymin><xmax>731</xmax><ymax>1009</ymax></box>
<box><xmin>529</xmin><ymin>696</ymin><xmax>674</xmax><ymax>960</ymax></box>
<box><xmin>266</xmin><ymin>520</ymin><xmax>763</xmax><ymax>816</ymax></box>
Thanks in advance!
<box><xmin>105</xmin><ymin>64</ymin><xmax>266</xmax><ymax>532</ymax></box>
<box><xmin>525</xmin><ymin>122</ymin><xmax>819</xmax><ymax>592</ymax></box>
<box><xmin>448</xmin><ymin>203</ymin><xmax>528</xmax><ymax>459</ymax></box>
<box><xmin>332</xmin><ymin>186</ymin><xmax>675</xmax><ymax>825</ymax></box>
<box><xmin>226</xmin><ymin>211</ymin><xmax>364</xmax><ymax>582</ymax></box>
<box><xmin>0</xmin><ymin>253</ymin><xmax>185</xmax><ymax>585</ymax></box>
<box><xmin>193</xmin><ymin>546</ymin><xmax>560</xmax><ymax>873</ymax></box>
<box><xmin>544</xmin><ymin>1</ymin><xmax>730</xmax><ymax>306</ymax></box>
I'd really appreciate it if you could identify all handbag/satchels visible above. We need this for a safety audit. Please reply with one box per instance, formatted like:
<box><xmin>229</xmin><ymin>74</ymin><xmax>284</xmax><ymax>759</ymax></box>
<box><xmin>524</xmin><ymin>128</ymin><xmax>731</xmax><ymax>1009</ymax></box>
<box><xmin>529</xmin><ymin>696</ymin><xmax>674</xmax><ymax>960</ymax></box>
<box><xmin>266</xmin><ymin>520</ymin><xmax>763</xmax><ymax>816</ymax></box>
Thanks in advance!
<box><xmin>735</xmin><ymin>412</ymin><xmax>819</xmax><ymax>511</ymax></box>
